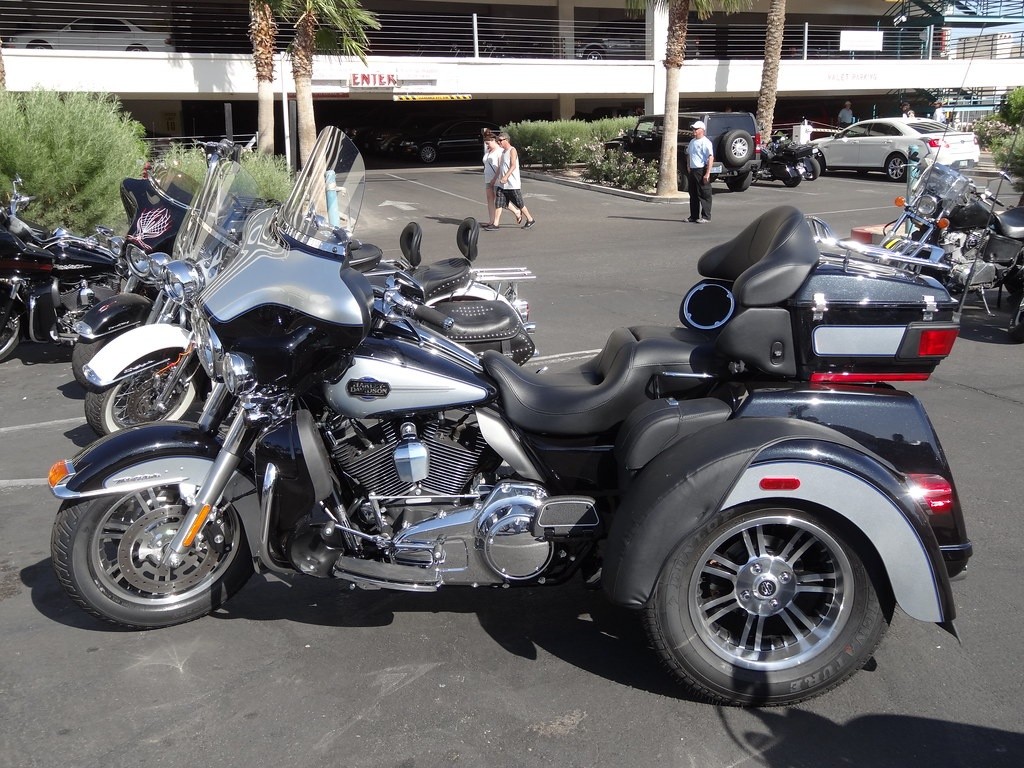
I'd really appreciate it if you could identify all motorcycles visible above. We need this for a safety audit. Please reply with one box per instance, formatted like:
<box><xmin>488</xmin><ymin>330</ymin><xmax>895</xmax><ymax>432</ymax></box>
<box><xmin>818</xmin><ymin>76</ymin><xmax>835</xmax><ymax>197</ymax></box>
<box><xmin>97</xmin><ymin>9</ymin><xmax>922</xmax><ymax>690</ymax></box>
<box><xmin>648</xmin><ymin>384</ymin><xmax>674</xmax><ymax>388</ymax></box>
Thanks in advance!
<box><xmin>49</xmin><ymin>124</ymin><xmax>977</xmax><ymax>708</ymax></box>
<box><xmin>1</xmin><ymin>131</ymin><xmax>541</xmax><ymax>440</ymax></box>
<box><xmin>751</xmin><ymin>129</ymin><xmax>822</xmax><ymax>188</ymax></box>
<box><xmin>876</xmin><ymin>141</ymin><xmax>1024</xmax><ymax>332</ymax></box>
<box><xmin>407</xmin><ymin>26</ymin><xmax>511</xmax><ymax>58</ymax></box>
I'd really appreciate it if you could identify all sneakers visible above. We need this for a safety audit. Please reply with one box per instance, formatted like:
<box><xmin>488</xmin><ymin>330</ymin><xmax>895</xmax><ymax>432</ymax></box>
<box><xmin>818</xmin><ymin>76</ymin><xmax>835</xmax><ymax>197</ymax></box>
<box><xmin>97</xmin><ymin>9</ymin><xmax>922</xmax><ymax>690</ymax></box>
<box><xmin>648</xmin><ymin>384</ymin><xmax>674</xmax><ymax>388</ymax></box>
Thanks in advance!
<box><xmin>484</xmin><ymin>223</ymin><xmax>499</xmax><ymax>231</ymax></box>
<box><xmin>521</xmin><ymin>218</ymin><xmax>536</xmax><ymax>229</ymax></box>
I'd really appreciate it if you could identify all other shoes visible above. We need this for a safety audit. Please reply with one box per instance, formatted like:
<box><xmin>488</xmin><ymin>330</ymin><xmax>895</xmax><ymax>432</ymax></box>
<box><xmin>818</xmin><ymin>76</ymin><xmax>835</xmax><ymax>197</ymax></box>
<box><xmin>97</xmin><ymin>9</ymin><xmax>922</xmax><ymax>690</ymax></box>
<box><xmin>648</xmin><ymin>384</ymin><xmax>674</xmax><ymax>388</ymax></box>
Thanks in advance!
<box><xmin>684</xmin><ymin>217</ymin><xmax>700</xmax><ymax>223</ymax></box>
<box><xmin>696</xmin><ymin>218</ymin><xmax>712</xmax><ymax>223</ymax></box>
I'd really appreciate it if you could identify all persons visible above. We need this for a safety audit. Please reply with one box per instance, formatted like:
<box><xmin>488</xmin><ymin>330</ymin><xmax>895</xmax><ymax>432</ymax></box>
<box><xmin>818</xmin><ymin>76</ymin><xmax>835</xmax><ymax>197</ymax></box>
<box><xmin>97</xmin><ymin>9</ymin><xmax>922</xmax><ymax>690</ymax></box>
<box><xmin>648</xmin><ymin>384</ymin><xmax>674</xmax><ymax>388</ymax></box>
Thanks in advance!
<box><xmin>683</xmin><ymin>121</ymin><xmax>714</xmax><ymax>223</ymax></box>
<box><xmin>481</xmin><ymin>129</ymin><xmax>535</xmax><ymax>231</ymax></box>
<box><xmin>838</xmin><ymin>101</ymin><xmax>852</xmax><ymax>128</ymax></box>
<box><xmin>900</xmin><ymin>102</ymin><xmax>914</xmax><ymax>118</ymax></box>
<box><xmin>932</xmin><ymin>102</ymin><xmax>946</xmax><ymax>123</ymax></box>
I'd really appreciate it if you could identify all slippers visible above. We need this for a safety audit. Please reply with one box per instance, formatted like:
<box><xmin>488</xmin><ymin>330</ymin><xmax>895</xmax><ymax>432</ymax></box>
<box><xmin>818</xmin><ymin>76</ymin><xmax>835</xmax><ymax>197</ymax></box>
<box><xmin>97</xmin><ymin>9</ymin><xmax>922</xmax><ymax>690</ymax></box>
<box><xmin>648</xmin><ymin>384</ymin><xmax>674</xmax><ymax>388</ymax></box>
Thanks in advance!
<box><xmin>481</xmin><ymin>222</ymin><xmax>493</xmax><ymax>228</ymax></box>
<box><xmin>517</xmin><ymin>211</ymin><xmax>522</xmax><ymax>224</ymax></box>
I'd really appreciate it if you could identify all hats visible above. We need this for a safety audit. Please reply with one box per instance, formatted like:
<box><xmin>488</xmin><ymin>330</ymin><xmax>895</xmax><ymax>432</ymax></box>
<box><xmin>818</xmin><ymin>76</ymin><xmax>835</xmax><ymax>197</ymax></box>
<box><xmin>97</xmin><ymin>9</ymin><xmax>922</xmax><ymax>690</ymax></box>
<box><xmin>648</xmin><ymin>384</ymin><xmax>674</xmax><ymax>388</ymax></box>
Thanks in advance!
<box><xmin>931</xmin><ymin>101</ymin><xmax>942</xmax><ymax>107</ymax></box>
<box><xmin>845</xmin><ymin>101</ymin><xmax>851</xmax><ymax>104</ymax></box>
<box><xmin>690</xmin><ymin>121</ymin><xmax>706</xmax><ymax>131</ymax></box>
<box><xmin>901</xmin><ymin>102</ymin><xmax>910</xmax><ymax>108</ymax></box>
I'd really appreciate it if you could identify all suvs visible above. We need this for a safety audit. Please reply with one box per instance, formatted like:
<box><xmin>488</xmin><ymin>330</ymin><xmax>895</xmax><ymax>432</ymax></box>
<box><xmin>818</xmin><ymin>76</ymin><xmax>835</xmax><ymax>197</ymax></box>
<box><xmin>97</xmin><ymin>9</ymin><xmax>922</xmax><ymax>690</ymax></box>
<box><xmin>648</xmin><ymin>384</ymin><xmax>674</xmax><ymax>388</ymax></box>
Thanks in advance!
<box><xmin>605</xmin><ymin>112</ymin><xmax>761</xmax><ymax>192</ymax></box>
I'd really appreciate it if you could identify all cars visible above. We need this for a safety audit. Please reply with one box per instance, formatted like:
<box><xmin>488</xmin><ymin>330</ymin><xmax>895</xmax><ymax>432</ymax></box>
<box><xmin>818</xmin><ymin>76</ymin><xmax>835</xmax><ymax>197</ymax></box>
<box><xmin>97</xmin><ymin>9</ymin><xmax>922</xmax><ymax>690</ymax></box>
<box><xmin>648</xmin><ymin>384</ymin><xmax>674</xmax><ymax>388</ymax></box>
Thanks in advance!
<box><xmin>5</xmin><ymin>15</ymin><xmax>179</xmax><ymax>53</ymax></box>
<box><xmin>394</xmin><ymin>119</ymin><xmax>501</xmax><ymax>163</ymax></box>
<box><xmin>807</xmin><ymin>117</ymin><xmax>981</xmax><ymax>183</ymax></box>
<box><xmin>377</xmin><ymin>133</ymin><xmax>402</xmax><ymax>153</ymax></box>
<box><xmin>574</xmin><ymin>19</ymin><xmax>699</xmax><ymax>60</ymax></box>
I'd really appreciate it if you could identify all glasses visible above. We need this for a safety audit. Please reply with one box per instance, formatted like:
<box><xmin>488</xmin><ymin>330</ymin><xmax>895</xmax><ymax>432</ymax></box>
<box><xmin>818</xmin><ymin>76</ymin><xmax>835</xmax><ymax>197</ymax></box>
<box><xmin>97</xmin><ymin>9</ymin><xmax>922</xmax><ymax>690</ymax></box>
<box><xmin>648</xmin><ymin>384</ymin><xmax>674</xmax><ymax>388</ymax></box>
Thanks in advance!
<box><xmin>495</xmin><ymin>138</ymin><xmax>507</xmax><ymax>142</ymax></box>
